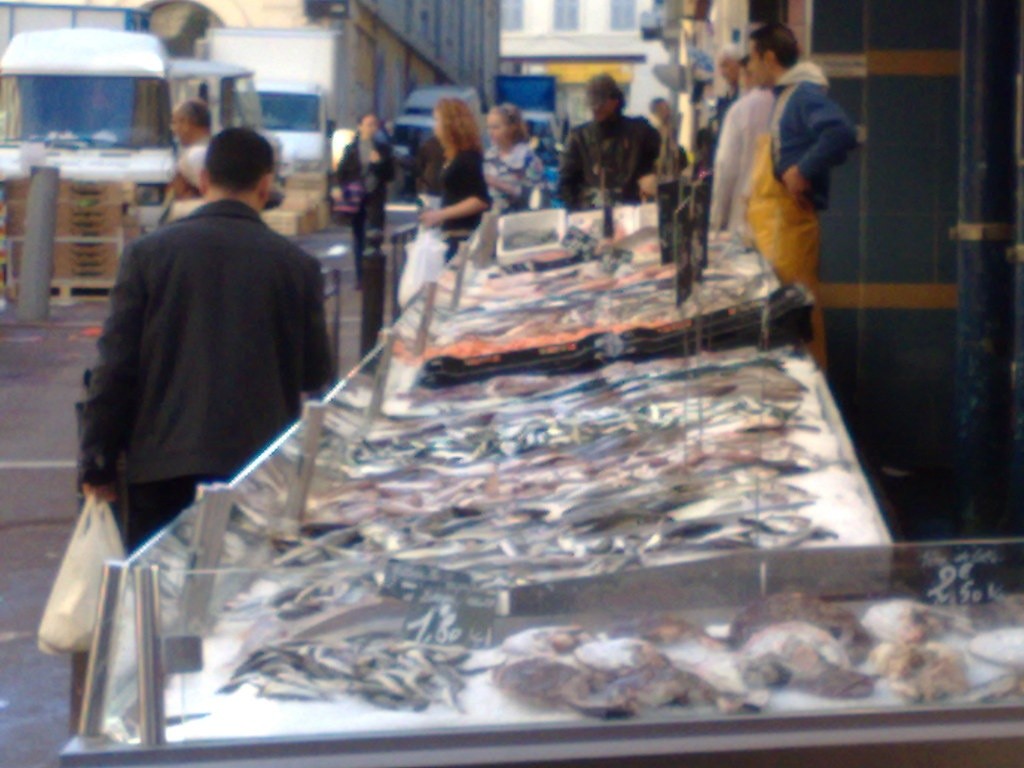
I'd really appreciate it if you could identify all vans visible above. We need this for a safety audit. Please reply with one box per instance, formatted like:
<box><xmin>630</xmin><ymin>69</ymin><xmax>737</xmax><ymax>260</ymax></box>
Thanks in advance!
<box><xmin>1</xmin><ymin>23</ymin><xmax>181</xmax><ymax>240</ymax></box>
<box><xmin>249</xmin><ymin>78</ymin><xmax>337</xmax><ymax>208</ymax></box>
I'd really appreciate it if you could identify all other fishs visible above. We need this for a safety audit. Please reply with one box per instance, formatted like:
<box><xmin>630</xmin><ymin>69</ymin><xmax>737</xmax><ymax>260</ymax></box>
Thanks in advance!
<box><xmin>216</xmin><ymin>231</ymin><xmax>1024</xmax><ymax>720</ymax></box>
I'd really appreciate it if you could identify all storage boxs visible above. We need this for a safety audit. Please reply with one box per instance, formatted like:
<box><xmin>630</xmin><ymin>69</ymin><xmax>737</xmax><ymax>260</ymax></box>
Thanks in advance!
<box><xmin>260</xmin><ymin>172</ymin><xmax>332</xmax><ymax>237</ymax></box>
<box><xmin>7</xmin><ymin>177</ymin><xmax>137</xmax><ymax>281</ymax></box>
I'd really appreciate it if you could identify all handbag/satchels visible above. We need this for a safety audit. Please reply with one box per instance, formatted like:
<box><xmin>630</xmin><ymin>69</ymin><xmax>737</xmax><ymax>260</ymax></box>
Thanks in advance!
<box><xmin>37</xmin><ymin>487</ymin><xmax>127</xmax><ymax>655</ymax></box>
<box><xmin>334</xmin><ymin>180</ymin><xmax>363</xmax><ymax>212</ymax></box>
<box><xmin>397</xmin><ymin>223</ymin><xmax>450</xmax><ymax>308</ymax></box>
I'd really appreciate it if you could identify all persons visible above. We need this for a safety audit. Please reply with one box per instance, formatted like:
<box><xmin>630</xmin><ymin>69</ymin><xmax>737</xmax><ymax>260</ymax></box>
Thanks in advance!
<box><xmin>331</xmin><ymin>49</ymin><xmax>773</xmax><ymax>290</ymax></box>
<box><xmin>739</xmin><ymin>21</ymin><xmax>858</xmax><ymax>376</ymax></box>
<box><xmin>160</xmin><ymin>101</ymin><xmax>215</xmax><ymax>233</ymax></box>
<box><xmin>75</xmin><ymin>126</ymin><xmax>334</xmax><ymax>564</ymax></box>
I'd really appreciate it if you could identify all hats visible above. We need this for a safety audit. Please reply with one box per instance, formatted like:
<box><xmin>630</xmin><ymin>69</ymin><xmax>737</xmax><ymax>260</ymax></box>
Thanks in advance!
<box><xmin>587</xmin><ymin>74</ymin><xmax>618</xmax><ymax>113</ymax></box>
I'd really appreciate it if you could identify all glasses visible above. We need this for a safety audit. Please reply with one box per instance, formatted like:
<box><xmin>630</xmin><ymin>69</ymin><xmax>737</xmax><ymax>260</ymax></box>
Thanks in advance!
<box><xmin>737</xmin><ymin>50</ymin><xmax>762</xmax><ymax>68</ymax></box>
